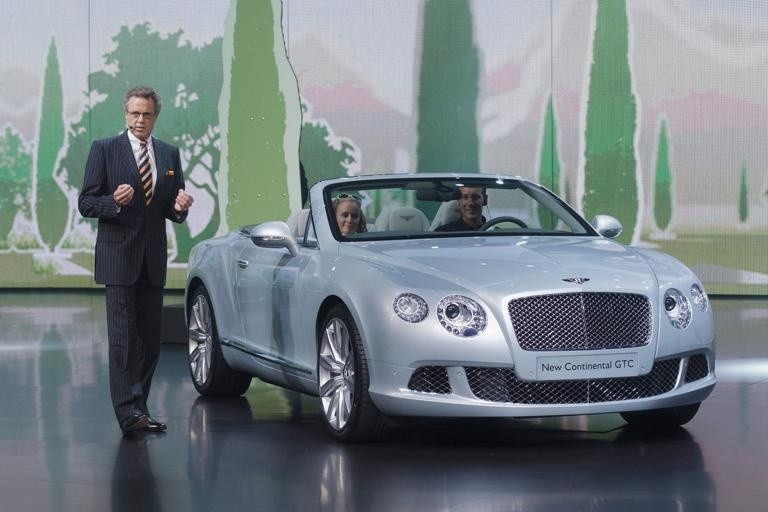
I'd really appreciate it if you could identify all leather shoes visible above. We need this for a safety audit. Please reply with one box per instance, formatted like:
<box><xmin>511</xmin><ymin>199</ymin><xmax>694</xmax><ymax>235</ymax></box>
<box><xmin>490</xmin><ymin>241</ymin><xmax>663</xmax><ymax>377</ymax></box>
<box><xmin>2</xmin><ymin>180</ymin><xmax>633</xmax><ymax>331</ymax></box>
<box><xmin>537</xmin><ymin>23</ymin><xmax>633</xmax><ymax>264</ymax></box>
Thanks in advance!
<box><xmin>123</xmin><ymin>412</ymin><xmax>148</xmax><ymax>433</ymax></box>
<box><xmin>144</xmin><ymin>414</ymin><xmax>167</xmax><ymax>431</ymax></box>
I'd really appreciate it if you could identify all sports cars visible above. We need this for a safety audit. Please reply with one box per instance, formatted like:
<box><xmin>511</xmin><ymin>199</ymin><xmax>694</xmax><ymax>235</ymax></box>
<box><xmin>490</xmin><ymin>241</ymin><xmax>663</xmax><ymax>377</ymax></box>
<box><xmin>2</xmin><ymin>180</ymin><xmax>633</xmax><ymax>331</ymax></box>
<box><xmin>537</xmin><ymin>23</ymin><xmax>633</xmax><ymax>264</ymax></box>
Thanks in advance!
<box><xmin>180</xmin><ymin>170</ymin><xmax>717</xmax><ymax>441</ymax></box>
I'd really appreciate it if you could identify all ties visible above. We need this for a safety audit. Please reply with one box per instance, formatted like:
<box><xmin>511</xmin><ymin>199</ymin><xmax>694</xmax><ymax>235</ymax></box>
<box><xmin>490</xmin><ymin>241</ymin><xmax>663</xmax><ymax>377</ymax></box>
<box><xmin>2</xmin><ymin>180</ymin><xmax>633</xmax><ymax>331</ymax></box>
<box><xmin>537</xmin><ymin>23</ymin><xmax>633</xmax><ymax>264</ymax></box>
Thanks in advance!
<box><xmin>135</xmin><ymin>140</ymin><xmax>153</xmax><ymax>205</ymax></box>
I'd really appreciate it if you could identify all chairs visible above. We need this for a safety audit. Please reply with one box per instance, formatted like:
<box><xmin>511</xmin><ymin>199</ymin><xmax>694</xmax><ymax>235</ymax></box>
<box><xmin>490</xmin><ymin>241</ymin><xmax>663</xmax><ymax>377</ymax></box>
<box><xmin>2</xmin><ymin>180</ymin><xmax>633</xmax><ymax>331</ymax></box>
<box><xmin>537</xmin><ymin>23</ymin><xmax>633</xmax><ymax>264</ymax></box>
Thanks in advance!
<box><xmin>432</xmin><ymin>200</ymin><xmax>462</xmax><ymax>231</ymax></box>
<box><xmin>374</xmin><ymin>206</ymin><xmax>429</xmax><ymax>232</ymax></box>
<box><xmin>297</xmin><ymin>208</ymin><xmax>318</xmax><ymax>239</ymax></box>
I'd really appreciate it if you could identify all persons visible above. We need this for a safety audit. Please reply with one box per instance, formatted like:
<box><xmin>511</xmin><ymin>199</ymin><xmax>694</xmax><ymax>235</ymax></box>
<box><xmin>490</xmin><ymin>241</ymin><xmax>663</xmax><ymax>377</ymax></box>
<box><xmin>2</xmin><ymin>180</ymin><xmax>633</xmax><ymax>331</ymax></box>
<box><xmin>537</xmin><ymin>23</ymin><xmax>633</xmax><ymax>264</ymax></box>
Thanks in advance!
<box><xmin>434</xmin><ymin>186</ymin><xmax>487</xmax><ymax>231</ymax></box>
<box><xmin>332</xmin><ymin>193</ymin><xmax>368</xmax><ymax>236</ymax></box>
<box><xmin>77</xmin><ymin>86</ymin><xmax>195</xmax><ymax>434</ymax></box>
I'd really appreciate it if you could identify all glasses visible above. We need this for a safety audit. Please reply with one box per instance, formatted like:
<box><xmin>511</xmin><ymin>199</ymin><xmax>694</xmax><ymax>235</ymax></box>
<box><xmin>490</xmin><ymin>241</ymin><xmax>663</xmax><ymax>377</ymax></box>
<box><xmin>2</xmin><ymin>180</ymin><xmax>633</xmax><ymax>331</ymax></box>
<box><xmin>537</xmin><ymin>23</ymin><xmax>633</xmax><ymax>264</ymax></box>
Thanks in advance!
<box><xmin>126</xmin><ymin>109</ymin><xmax>157</xmax><ymax>120</ymax></box>
<box><xmin>335</xmin><ymin>193</ymin><xmax>362</xmax><ymax>202</ymax></box>
